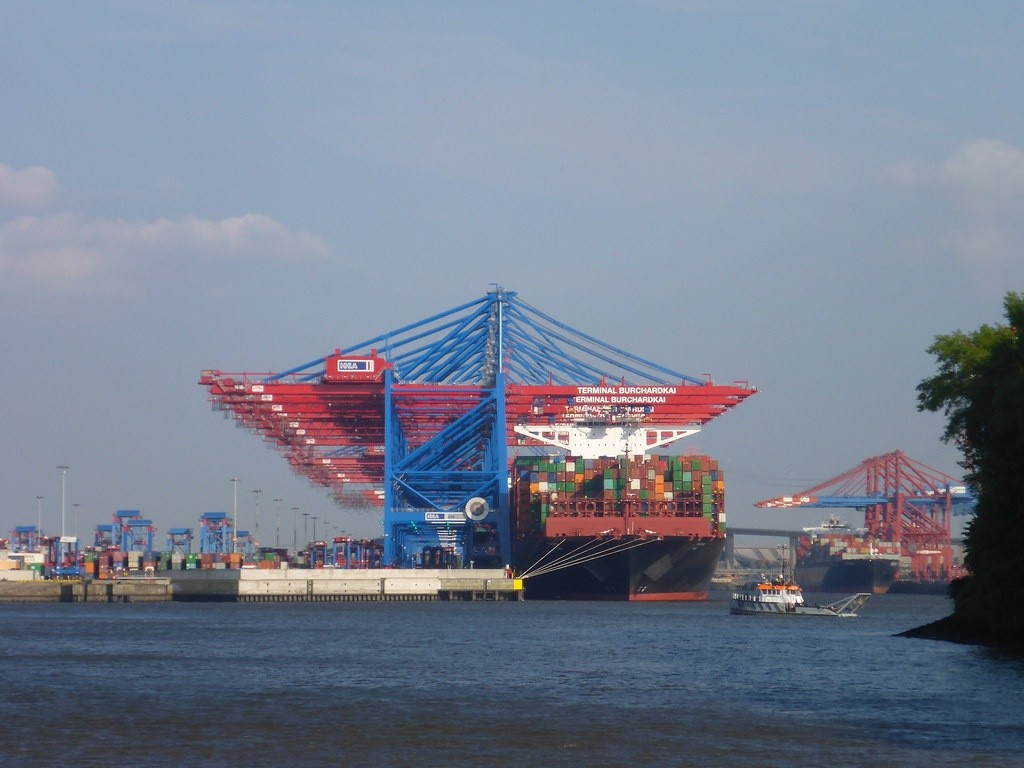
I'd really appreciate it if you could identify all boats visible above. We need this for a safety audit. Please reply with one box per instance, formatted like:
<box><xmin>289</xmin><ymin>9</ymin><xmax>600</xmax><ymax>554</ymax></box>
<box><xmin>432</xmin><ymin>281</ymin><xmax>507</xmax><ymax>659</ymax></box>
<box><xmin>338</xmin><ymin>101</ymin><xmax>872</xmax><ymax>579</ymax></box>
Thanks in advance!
<box><xmin>727</xmin><ymin>544</ymin><xmax>872</xmax><ymax>618</ymax></box>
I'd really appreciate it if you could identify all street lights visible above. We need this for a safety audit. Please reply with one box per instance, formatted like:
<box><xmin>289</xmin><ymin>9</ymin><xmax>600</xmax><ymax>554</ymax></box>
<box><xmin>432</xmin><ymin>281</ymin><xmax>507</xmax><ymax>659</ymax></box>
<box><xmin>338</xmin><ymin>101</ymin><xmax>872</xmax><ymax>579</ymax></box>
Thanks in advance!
<box><xmin>324</xmin><ymin>522</ymin><xmax>330</xmax><ymax>542</ymax></box>
<box><xmin>310</xmin><ymin>517</ymin><xmax>319</xmax><ymax>543</ymax></box>
<box><xmin>332</xmin><ymin>526</ymin><xmax>338</xmax><ymax>539</ymax></box>
<box><xmin>251</xmin><ymin>489</ymin><xmax>262</xmax><ymax>546</ymax></box>
<box><xmin>55</xmin><ymin>464</ymin><xmax>73</xmax><ymax>561</ymax></box>
<box><xmin>291</xmin><ymin>506</ymin><xmax>300</xmax><ymax>555</ymax></box>
<box><xmin>301</xmin><ymin>514</ymin><xmax>309</xmax><ymax>546</ymax></box>
<box><xmin>228</xmin><ymin>478</ymin><xmax>242</xmax><ymax>553</ymax></box>
<box><xmin>34</xmin><ymin>495</ymin><xmax>45</xmax><ymax>538</ymax></box>
<box><xmin>272</xmin><ymin>497</ymin><xmax>283</xmax><ymax>548</ymax></box>
<box><xmin>340</xmin><ymin>530</ymin><xmax>346</xmax><ymax>536</ymax></box>
<box><xmin>72</xmin><ymin>503</ymin><xmax>81</xmax><ymax>537</ymax></box>
